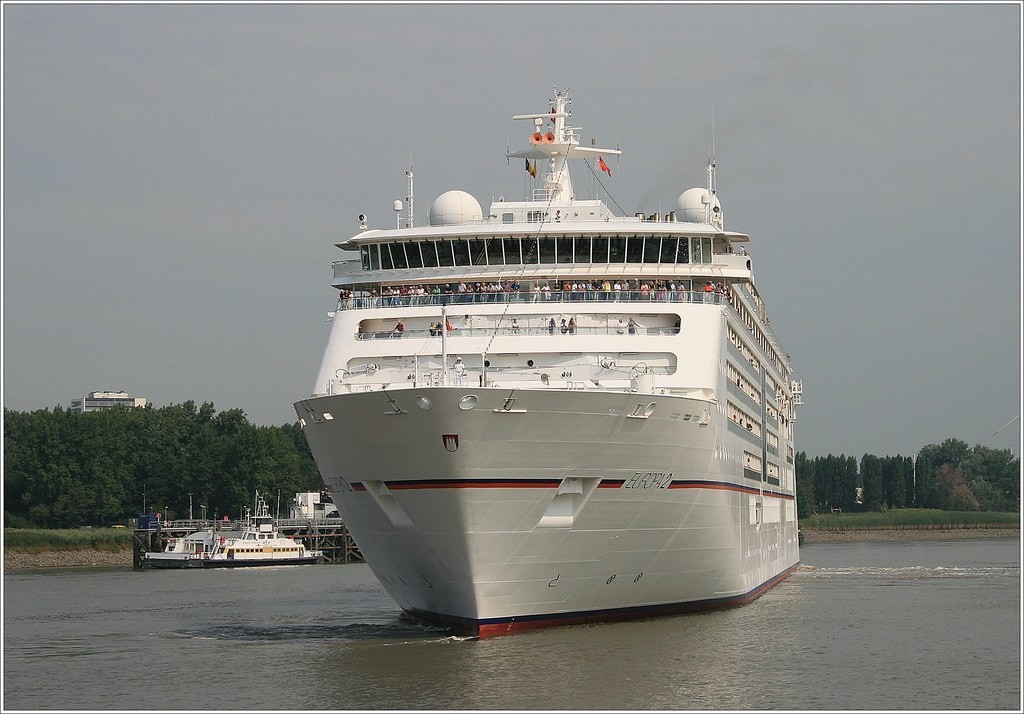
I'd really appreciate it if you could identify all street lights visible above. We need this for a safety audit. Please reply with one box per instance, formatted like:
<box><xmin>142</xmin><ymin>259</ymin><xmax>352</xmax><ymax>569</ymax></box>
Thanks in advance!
<box><xmin>150</xmin><ymin>506</ymin><xmax>154</xmax><ymax>514</ymax></box>
<box><xmin>142</xmin><ymin>494</ymin><xmax>145</xmax><ymax>514</ymax></box>
<box><xmin>188</xmin><ymin>492</ymin><xmax>195</xmax><ymax>519</ymax></box>
<box><xmin>243</xmin><ymin>506</ymin><xmax>247</xmax><ymax>523</ymax></box>
<box><xmin>200</xmin><ymin>504</ymin><xmax>206</xmax><ymax>519</ymax></box>
<box><xmin>165</xmin><ymin>506</ymin><xmax>168</xmax><ymax>521</ymax></box>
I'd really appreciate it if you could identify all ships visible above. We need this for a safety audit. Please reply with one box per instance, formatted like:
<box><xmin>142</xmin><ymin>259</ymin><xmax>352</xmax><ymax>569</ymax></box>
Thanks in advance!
<box><xmin>288</xmin><ymin>84</ymin><xmax>808</xmax><ymax>642</ymax></box>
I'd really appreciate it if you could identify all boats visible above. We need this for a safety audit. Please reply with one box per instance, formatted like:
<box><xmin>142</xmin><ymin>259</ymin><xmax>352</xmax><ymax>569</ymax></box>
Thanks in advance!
<box><xmin>143</xmin><ymin>528</ymin><xmax>242</xmax><ymax>569</ymax></box>
<box><xmin>202</xmin><ymin>489</ymin><xmax>324</xmax><ymax>567</ymax></box>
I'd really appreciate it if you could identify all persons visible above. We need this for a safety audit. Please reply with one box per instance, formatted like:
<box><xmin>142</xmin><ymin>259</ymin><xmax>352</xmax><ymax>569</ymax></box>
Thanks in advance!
<box><xmin>556</xmin><ymin>210</ymin><xmax>561</xmax><ymax>222</ymax></box>
<box><xmin>614</xmin><ymin>281</ymin><xmax>621</xmax><ymax>302</ymax></box>
<box><xmin>642</xmin><ymin>276</ymin><xmax>688</xmax><ymax>303</ymax></box>
<box><xmin>747</xmin><ymin>323</ymin><xmax>752</xmax><ymax>332</ymax></box>
<box><xmin>705</xmin><ymin>281</ymin><xmax>731</xmax><ymax>300</ymax></box>
<box><xmin>621</xmin><ymin>279</ymin><xmax>630</xmax><ymax>300</ymax></box>
<box><xmin>444</xmin><ymin>284</ymin><xmax>453</xmax><ymax>303</ymax></box>
<box><xmin>462</xmin><ymin>315</ymin><xmax>471</xmax><ymax>334</ymax></box>
<box><xmin>453</xmin><ymin>357</ymin><xmax>465</xmax><ymax>378</ymax></box>
<box><xmin>534</xmin><ymin>282</ymin><xmax>560</xmax><ymax>301</ymax></box>
<box><xmin>429</xmin><ymin>321</ymin><xmax>443</xmax><ymax>336</ymax></box>
<box><xmin>550</xmin><ymin>317</ymin><xmax>576</xmax><ymax>334</ymax></box>
<box><xmin>458</xmin><ymin>279</ymin><xmax>520</xmax><ymax>302</ymax></box>
<box><xmin>616</xmin><ymin>317</ymin><xmax>640</xmax><ymax>335</ymax></box>
<box><xmin>383</xmin><ymin>285</ymin><xmax>441</xmax><ymax>305</ymax></box>
<box><xmin>394</xmin><ymin>321</ymin><xmax>404</xmax><ymax>335</ymax></box>
<box><xmin>370</xmin><ymin>287</ymin><xmax>379</xmax><ymax>305</ymax></box>
<box><xmin>630</xmin><ymin>278</ymin><xmax>640</xmax><ymax>300</ymax></box>
<box><xmin>739</xmin><ymin>245</ymin><xmax>748</xmax><ymax>256</ymax></box>
<box><xmin>512</xmin><ymin>319</ymin><xmax>519</xmax><ymax>328</ymax></box>
<box><xmin>339</xmin><ymin>289</ymin><xmax>352</xmax><ymax>307</ymax></box>
<box><xmin>564</xmin><ymin>278</ymin><xmax>611</xmax><ymax>300</ymax></box>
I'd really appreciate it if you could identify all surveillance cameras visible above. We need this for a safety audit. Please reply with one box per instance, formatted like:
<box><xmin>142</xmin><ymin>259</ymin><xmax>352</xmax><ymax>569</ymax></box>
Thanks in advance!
<box><xmin>357</xmin><ymin>214</ymin><xmax>368</xmax><ymax>223</ymax></box>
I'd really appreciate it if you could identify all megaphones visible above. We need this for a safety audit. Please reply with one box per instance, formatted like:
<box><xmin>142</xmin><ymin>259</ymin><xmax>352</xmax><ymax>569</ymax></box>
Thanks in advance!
<box><xmin>531</xmin><ymin>132</ymin><xmax>555</xmax><ymax>144</ymax></box>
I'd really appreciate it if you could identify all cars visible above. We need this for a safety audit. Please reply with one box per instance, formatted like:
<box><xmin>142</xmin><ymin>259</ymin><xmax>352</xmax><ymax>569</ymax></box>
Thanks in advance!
<box><xmin>325</xmin><ymin>509</ymin><xmax>340</xmax><ymax>518</ymax></box>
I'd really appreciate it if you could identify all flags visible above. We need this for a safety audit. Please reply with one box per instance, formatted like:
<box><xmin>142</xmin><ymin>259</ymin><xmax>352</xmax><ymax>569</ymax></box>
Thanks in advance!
<box><xmin>551</xmin><ymin>107</ymin><xmax>556</xmax><ymax>123</ymax></box>
<box><xmin>600</xmin><ymin>157</ymin><xmax>611</xmax><ymax>177</ymax></box>
<box><xmin>525</xmin><ymin>158</ymin><xmax>536</xmax><ymax>177</ymax></box>
